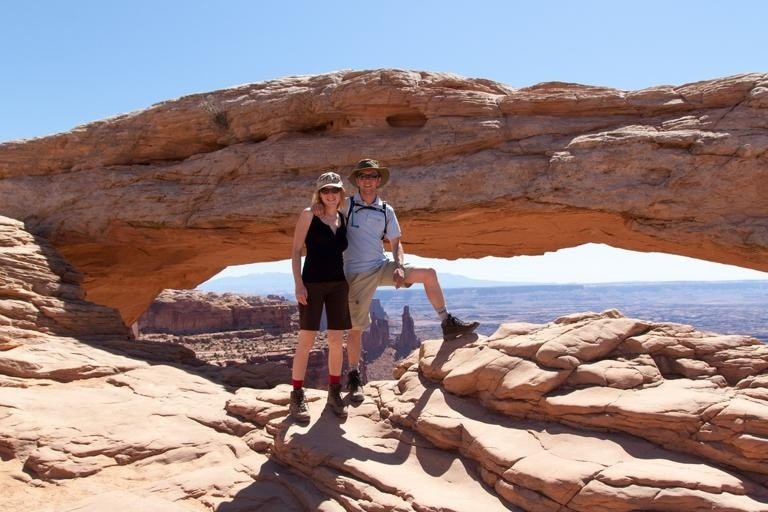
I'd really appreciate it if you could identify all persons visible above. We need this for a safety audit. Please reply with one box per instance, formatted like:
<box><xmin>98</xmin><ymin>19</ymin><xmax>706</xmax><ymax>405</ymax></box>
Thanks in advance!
<box><xmin>312</xmin><ymin>157</ymin><xmax>479</xmax><ymax>403</ymax></box>
<box><xmin>289</xmin><ymin>170</ymin><xmax>348</xmax><ymax>423</ymax></box>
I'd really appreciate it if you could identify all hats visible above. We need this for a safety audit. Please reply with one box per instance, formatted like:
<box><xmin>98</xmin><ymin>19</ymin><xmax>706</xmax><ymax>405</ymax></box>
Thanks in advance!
<box><xmin>316</xmin><ymin>171</ymin><xmax>345</xmax><ymax>192</ymax></box>
<box><xmin>346</xmin><ymin>158</ymin><xmax>391</xmax><ymax>189</ymax></box>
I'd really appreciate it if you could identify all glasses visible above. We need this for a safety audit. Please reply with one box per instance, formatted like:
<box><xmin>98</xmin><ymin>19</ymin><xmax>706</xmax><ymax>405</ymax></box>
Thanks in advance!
<box><xmin>356</xmin><ymin>171</ymin><xmax>379</xmax><ymax>179</ymax></box>
<box><xmin>320</xmin><ymin>187</ymin><xmax>341</xmax><ymax>194</ymax></box>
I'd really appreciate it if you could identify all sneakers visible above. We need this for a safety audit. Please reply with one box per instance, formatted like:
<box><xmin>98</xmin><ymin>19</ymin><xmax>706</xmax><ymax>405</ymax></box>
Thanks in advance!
<box><xmin>440</xmin><ymin>314</ymin><xmax>480</xmax><ymax>341</ymax></box>
<box><xmin>348</xmin><ymin>369</ymin><xmax>366</xmax><ymax>402</ymax></box>
<box><xmin>289</xmin><ymin>388</ymin><xmax>311</xmax><ymax>423</ymax></box>
<box><xmin>326</xmin><ymin>383</ymin><xmax>349</xmax><ymax>418</ymax></box>
